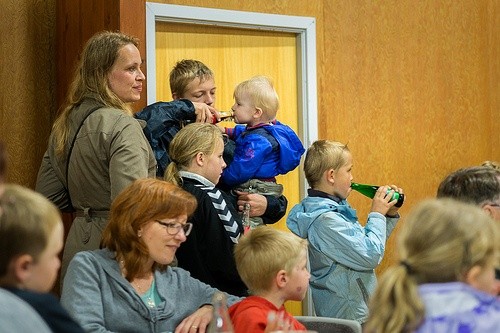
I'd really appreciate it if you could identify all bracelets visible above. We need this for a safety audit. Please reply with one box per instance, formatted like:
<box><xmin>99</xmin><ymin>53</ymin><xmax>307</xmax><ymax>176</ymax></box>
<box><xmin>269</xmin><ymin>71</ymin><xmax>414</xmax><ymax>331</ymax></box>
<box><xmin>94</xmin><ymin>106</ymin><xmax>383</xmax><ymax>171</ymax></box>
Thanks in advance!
<box><xmin>385</xmin><ymin>213</ymin><xmax>397</xmax><ymax>218</ymax></box>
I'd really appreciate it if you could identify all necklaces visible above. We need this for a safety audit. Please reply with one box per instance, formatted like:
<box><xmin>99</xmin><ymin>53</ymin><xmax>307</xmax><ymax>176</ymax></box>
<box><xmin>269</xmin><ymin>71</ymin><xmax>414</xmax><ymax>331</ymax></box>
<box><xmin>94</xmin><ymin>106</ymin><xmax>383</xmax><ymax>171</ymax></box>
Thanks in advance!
<box><xmin>115</xmin><ymin>252</ymin><xmax>156</xmax><ymax>308</ymax></box>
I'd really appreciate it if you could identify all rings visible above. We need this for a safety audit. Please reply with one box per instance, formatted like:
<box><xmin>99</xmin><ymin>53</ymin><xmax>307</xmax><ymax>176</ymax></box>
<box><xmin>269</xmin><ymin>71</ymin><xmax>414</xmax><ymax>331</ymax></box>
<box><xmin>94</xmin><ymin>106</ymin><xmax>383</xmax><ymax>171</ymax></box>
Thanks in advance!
<box><xmin>191</xmin><ymin>325</ymin><xmax>198</xmax><ymax>329</ymax></box>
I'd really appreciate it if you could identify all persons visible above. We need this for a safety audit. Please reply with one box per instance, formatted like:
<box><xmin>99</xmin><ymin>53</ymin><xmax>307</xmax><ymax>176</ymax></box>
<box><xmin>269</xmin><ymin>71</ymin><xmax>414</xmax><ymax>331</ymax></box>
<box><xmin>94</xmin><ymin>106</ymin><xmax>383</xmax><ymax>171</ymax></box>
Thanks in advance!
<box><xmin>163</xmin><ymin>122</ymin><xmax>253</xmax><ymax>298</ymax></box>
<box><xmin>437</xmin><ymin>161</ymin><xmax>500</xmax><ymax>299</ymax></box>
<box><xmin>215</xmin><ymin>76</ymin><xmax>306</xmax><ymax>235</ymax></box>
<box><xmin>133</xmin><ymin>58</ymin><xmax>288</xmax><ymax>224</ymax></box>
<box><xmin>35</xmin><ymin>30</ymin><xmax>158</xmax><ymax>300</ymax></box>
<box><xmin>60</xmin><ymin>175</ymin><xmax>247</xmax><ymax>333</ymax></box>
<box><xmin>362</xmin><ymin>197</ymin><xmax>500</xmax><ymax>333</ymax></box>
<box><xmin>221</xmin><ymin>225</ymin><xmax>312</xmax><ymax>333</ymax></box>
<box><xmin>0</xmin><ymin>181</ymin><xmax>64</xmax><ymax>294</ymax></box>
<box><xmin>286</xmin><ymin>138</ymin><xmax>406</xmax><ymax>333</ymax></box>
<box><xmin>0</xmin><ymin>283</ymin><xmax>85</xmax><ymax>333</ymax></box>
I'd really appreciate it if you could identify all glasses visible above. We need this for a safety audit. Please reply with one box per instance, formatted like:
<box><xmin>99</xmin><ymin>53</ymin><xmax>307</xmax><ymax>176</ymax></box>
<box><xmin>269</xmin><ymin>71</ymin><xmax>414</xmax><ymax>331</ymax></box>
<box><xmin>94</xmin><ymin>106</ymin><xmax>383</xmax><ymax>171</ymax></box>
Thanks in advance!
<box><xmin>155</xmin><ymin>220</ymin><xmax>192</xmax><ymax>236</ymax></box>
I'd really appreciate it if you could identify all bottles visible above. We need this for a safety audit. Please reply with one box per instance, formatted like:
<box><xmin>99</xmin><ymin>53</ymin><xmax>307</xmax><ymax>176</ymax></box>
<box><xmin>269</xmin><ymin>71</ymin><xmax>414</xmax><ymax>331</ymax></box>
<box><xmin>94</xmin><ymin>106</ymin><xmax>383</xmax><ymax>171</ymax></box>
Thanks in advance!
<box><xmin>350</xmin><ymin>183</ymin><xmax>404</xmax><ymax>208</ymax></box>
<box><xmin>207</xmin><ymin>291</ymin><xmax>234</xmax><ymax>333</ymax></box>
<box><xmin>241</xmin><ymin>204</ymin><xmax>250</xmax><ymax>234</ymax></box>
<box><xmin>185</xmin><ymin>111</ymin><xmax>235</xmax><ymax>125</ymax></box>
